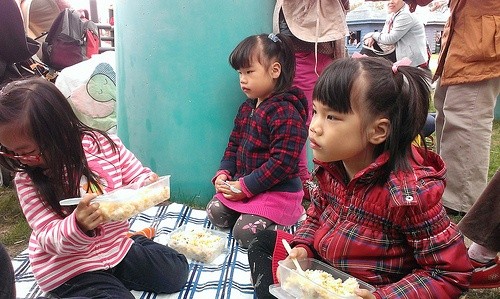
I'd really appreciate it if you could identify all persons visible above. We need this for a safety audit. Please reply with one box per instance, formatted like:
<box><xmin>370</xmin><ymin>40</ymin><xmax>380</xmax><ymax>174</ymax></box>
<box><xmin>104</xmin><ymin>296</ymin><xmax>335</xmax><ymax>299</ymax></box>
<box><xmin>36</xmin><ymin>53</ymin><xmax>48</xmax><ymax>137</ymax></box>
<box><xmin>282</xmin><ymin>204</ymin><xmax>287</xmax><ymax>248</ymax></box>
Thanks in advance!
<box><xmin>0</xmin><ymin>0</ymin><xmax>190</xmax><ymax>299</ymax></box>
<box><xmin>205</xmin><ymin>0</ymin><xmax>500</xmax><ymax>299</ymax></box>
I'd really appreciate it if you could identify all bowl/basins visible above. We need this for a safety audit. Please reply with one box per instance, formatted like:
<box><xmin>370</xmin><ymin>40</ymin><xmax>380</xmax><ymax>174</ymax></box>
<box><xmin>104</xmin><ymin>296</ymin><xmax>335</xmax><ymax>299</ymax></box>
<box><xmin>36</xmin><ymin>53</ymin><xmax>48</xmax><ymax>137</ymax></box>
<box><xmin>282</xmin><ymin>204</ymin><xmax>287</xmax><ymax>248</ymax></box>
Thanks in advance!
<box><xmin>277</xmin><ymin>258</ymin><xmax>377</xmax><ymax>299</ymax></box>
<box><xmin>167</xmin><ymin>222</ymin><xmax>228</xmax><ymax>264</ymax></box>
<box><xmin>87</xmin><ymin>174</ymin><xmax>172</xmax><ymax>224</ymax></box>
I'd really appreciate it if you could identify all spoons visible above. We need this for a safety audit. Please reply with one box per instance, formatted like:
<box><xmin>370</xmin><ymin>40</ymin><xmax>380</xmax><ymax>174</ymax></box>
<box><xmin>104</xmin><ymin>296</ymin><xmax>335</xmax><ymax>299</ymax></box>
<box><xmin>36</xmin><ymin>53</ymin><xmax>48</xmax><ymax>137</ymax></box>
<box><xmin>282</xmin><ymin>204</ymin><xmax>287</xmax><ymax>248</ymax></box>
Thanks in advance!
<box><xmin>60</xmin><ymin>189</ymin><xmax>132</xmax><ymax>205</ymax></box>
<box><xmin>220</xmin><ymin>179</ymin><xmax>241</xmax><ymax>194</ymax></box>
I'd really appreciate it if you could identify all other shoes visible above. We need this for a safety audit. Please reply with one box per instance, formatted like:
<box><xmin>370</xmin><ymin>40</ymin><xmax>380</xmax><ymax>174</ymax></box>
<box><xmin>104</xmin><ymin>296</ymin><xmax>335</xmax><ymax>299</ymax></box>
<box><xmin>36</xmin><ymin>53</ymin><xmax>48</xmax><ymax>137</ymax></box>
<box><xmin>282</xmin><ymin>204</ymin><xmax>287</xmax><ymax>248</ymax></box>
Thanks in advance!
<box><xmin>467</xmin><ymin>248</ymin><xmax>500</xmax><ymax>288</ymax></box>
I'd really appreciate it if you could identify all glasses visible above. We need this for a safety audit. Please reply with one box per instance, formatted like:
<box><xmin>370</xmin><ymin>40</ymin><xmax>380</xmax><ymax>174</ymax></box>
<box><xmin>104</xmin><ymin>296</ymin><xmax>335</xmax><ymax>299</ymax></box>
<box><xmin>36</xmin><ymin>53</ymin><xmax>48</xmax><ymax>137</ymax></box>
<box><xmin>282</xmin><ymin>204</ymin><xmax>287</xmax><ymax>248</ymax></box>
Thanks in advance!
<box><xmin>0</xmin><ymin>146</ymin><xmax>43</xmax><ymax>162</ymax></box>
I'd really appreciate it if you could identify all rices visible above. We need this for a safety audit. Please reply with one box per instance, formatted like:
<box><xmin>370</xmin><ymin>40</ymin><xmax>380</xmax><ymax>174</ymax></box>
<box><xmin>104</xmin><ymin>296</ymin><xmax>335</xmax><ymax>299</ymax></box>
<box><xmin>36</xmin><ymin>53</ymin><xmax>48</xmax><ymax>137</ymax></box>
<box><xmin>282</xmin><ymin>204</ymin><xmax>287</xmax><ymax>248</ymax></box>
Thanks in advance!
<box><xmin>93</xmin><ymin>184</ymin><xmax>169</xmax><ymax>220</ymax></box>
<box><xmin>283</xmin><ymin>268</ymin><xmax>360</xmax><ymax>299</ymax></box>
<box><xmin>169</xmin><ymin>227</ymin><xmax>224</xmax><ymax>264</ymax></box>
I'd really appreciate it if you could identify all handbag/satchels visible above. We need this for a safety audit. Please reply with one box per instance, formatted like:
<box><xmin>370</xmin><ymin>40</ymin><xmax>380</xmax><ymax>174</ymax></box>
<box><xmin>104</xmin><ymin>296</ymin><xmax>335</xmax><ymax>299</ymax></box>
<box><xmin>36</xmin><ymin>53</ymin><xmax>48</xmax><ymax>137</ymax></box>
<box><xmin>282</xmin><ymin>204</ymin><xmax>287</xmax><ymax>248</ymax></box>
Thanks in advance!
<box><xmin>361</xmin><ymin>32</ymin><xmax>396</xmax><ymax>55</ymax></box>
<box><xmin>47</xmin><ymin>35</ymin><xmax>84</xmax><ymax>68</ymax></box>
